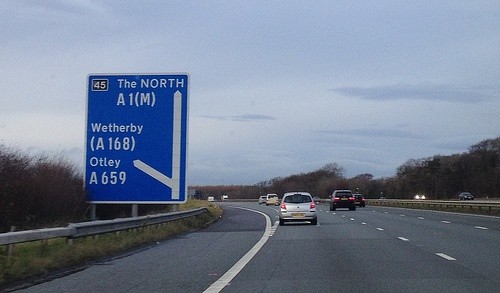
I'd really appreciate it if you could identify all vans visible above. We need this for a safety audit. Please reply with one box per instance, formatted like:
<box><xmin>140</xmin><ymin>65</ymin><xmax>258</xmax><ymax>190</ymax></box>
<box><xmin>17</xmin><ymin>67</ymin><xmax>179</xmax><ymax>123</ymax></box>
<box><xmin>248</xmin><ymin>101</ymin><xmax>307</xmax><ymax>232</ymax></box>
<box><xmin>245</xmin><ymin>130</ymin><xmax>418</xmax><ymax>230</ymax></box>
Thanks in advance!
<box><xmin>266</xmin><ymin>194</ymin><xmax>279</xmax><ymax>206</ymax></box>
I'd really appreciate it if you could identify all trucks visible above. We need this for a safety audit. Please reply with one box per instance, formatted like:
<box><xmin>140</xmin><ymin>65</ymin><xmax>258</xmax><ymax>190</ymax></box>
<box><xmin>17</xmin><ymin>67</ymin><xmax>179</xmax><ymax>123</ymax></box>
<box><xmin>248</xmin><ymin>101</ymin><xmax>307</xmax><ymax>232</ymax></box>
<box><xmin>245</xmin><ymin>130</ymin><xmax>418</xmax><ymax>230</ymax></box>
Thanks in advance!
<box><xmin>221</xmin><ymin>194</ymin><xmax>229</xmax><ymax>200</ymax></box>
<box><xmin>208</xmin><ymin>196</ymin><xmax>214</xmax><ymax>202</ymax></box>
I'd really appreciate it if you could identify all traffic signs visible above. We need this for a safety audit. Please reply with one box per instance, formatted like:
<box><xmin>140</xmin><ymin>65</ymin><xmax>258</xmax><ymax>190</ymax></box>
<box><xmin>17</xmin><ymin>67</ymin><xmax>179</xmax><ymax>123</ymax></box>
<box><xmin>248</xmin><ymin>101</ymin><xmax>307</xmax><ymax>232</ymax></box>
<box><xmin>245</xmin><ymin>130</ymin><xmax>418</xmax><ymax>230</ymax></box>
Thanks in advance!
<box><xmin>85</xmin><ymin>72</ymin><xmax>189</xmax><ymax>204</ymax></box>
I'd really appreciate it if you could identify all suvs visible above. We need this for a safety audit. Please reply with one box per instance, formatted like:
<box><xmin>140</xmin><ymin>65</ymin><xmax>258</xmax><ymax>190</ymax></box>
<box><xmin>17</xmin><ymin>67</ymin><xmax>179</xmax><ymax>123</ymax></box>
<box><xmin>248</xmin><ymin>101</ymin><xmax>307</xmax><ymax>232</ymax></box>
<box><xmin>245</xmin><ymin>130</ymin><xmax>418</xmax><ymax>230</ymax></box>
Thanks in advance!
<box><xmin>353</xmin><ymin>193</ymin><xmax>366</xmax><ymax>207</ymax></box>
<box><xmin>329</xmin><ymin>188</ymin><xmax>356</xmax><ymax>211</ymax></box>
<box><xmin>258</xmin><ymin>196</ymin><xmax>267</xmax><ymax>205</ymax></box>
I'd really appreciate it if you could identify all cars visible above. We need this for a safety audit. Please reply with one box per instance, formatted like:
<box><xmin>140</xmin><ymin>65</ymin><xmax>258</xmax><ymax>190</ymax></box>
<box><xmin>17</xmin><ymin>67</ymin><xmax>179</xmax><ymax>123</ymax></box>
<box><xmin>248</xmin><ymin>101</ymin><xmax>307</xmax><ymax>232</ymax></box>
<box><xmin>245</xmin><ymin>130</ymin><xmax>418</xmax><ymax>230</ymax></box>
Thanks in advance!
<box><xmin>279</xmin><ymin>190</ymin><xmax>317</xmax><ymax>226</ymax></box>
<box><xmin>312</xmin><ymin>196</ymin><xmax>321</xmax><ymax>204</ymax></box>
<box><xmin>458</xmin><ymin>192</ymin><xmax>474</xmax><ymax>201</ymax></box>
